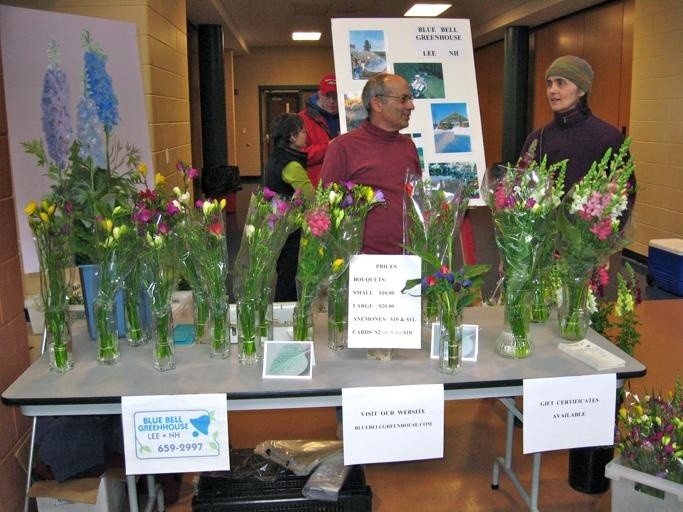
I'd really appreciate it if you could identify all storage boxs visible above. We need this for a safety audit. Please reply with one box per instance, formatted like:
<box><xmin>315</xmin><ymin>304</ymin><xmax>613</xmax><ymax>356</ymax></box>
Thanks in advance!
<box><xmin>14</xmin><ymin>430</ymin><xmax>140</xmax><ymax>512</ymax></box>
<box><xmin>645</xmin><ymin>238</ymin><xmax>683</xmax><ymax>297</ymax></box>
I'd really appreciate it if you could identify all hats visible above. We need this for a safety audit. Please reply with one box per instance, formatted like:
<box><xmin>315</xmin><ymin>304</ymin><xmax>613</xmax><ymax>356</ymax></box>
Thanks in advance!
<box><xmin>319</xmin><ymin>72</ymin><xmax>339</xmax><ymax>93</ymax></box>
<box><xmin>545</xmin><ymin>56</ymin><xmax>594</xmax><ymax>94</ymax></box>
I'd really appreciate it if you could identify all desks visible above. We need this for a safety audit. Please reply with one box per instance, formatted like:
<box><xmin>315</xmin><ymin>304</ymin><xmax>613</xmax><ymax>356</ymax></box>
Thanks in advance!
<box><xmin>0</xmin><ymin>301</ymin><xmax>647</xmax><ymax>512</ymax></box>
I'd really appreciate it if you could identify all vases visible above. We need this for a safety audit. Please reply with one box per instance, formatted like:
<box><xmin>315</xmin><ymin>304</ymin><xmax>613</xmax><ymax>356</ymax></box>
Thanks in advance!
<box><xmin>493</xmin><ymin>279</ymin><xmax>537</xmax><ymax>359</ymax></box>
<box><xmin>568</xmin><ymin>378</ymin><xmax>625</xmax><ymax>494</ymax></box>
<box><xmin>93</xmin><ymin>297</ymin><xmax>121</xmax><ymax>366</ymax></box>
<box><xmin>42</xmin><ymin>305</ymin><xmax>74</xmax><ymax>374</ymax></box>
<box><xmin>528</xmin><ymin>268</ymin><xmax>553</xmax><ymax>324</ymax></box>
<box><xmin>328</xmin><ymin>287</ymin><xmax>347</xmax><ymax>352</ymax></box>
<box><xmin>604</xmin><ymin>451</ymin><xmax>683</xmax><ymax>512</ymax></box>
<box><xmin>77</xmin><ymin>262</ymin><xmax>155</xmax><ymax>348</ymax></box>
<box><xmin>236</xmin><ymin>286</ymin><xmax>273</xmax><ymax>367</ymax></box>
<box><xmin>421</xmin><ymin>294</ymin><xmax>442</xmax><ymax>330</ymax></box>
<box><xmin>439</xmin><ymin>304</ymin><xmax>464</xmax><ymax>376</ymax></box>
<box><xmin>192</xmin><ymin>291</ymin><xmax>231</xmax><ymax>360</ymax></box>
<box><xmin>557</xmin><ymin>263</ymin><xmax>594</xmax><ymax>341</ymax></box>
<box><xmin>151</xmin><ymin>303</ymin><xmax>176</xmax><ymax>372</ymax></box>
<box><xmin>293</xmin><ymin>307</ymin><xmax>314</xmax><ymax>341</ymax></box>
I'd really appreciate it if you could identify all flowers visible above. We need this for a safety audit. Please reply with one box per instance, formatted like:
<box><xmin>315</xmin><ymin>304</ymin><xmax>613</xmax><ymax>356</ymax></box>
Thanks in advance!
<box><xmin>24</xmin><ymin>28</ymin><xmax>124</xmax><ymax>183</ymax></box>
<box><xmin>489</xmin><ymin>135</ymin><xmax>646</xmax><ymax>358</ymax></box>
<box><xmin>616</xmin><ymin>378</ymin><xmax>682</xmax><ymax>498</ymax></box>
<box><xmin>395</xmin><ymin>177</ymin><xmax>492</xmax><ymax>369</ymax></box>
<box><xmin>232</xmin><ymin>184</ymin><xmax>306</xmax><ymax>356</ymax></box>
<box><xmin>292</xmin><ymin>177</ymin><xmax>388</xmax><ymax>341</ymax></box>
<box><xmin>19</xmin><ymin>115</ymin><xmax>229</xmax><ymax>368</ymax></box>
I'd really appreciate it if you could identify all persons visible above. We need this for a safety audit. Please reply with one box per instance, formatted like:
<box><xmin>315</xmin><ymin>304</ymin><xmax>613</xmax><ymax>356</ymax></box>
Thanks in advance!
<box><xmin>295</xmin><ymin>74</ymin><xmax>339</xmax><ymax>188</ymax></box>
<box><xmin>515</xmin><ymin>55</ymin><xmax>638</xmax><ymax>301</ymax></box>
<box><xmin>314</xmin><ymin>73</ymin><xmax>430</xmax><ymax>255</ymax></box>
<box><xmin>263</xmin><ymin>110</ymin><xmax>315</xmax><ymax>303</ymax></box>
<box><xmin>491</xmin><ymin>163</ymin><xmax>513</xmax><ymax>281</ymax></box>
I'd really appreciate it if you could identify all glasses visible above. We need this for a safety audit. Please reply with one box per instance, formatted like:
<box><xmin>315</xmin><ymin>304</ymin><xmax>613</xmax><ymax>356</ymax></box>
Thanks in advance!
<box><xmin>374</xmin><ymin>92</ymin><xmax>413</xmax><ymax>105</ymax></box>
